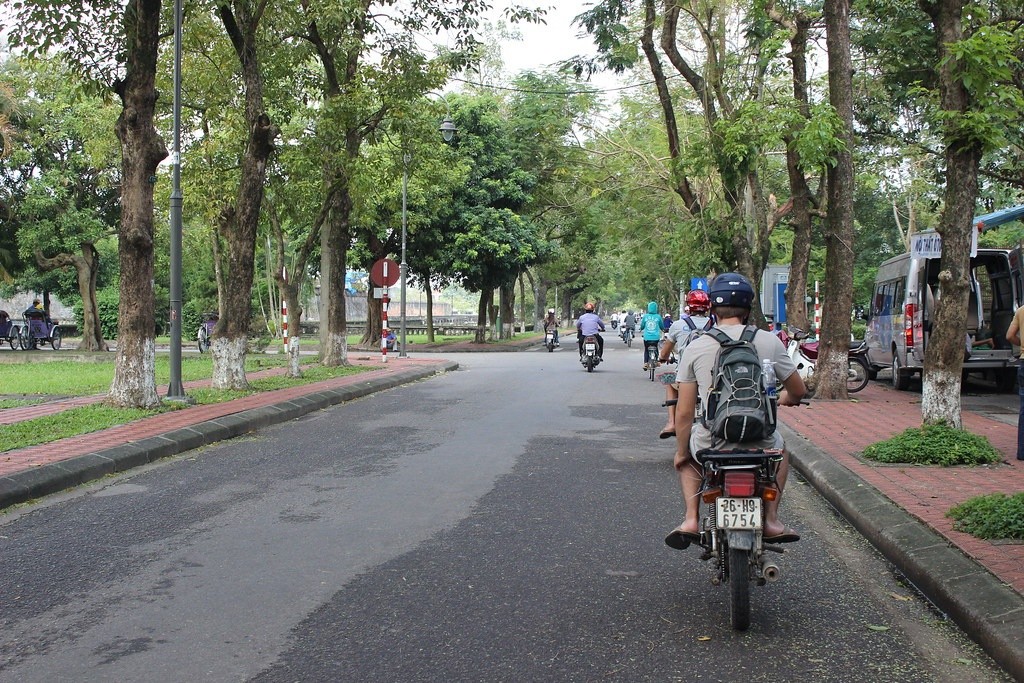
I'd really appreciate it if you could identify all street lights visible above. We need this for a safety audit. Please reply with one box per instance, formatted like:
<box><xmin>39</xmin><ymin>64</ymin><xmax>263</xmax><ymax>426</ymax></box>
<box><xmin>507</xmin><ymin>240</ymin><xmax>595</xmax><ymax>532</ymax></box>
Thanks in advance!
<box><xmin>397</xmin><ymin>90</ymin><xmax>460</xmax><ymax>357</ymax></box>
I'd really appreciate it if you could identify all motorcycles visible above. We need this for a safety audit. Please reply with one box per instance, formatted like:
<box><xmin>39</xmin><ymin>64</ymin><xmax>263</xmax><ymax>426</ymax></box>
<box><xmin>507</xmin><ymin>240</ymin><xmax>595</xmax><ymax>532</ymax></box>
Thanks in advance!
<box><xmin>783</xmin><ymin>320</ymin><xmax>874</xmax><ymax>393</ymax></box>
<box><xmin>662</xmin><ymin>383</ymin><xmax>817</xmax><ymax>633</ymax></box>
<box><xmin>576</xmin><ymin>328</ymin><xmax>605</xmax><ymax>372</ymax></box>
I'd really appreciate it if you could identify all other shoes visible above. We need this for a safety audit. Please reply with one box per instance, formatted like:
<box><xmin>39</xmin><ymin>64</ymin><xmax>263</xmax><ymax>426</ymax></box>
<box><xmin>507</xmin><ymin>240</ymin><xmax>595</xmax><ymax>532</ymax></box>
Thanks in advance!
<box><xmin>643</xmin><ymin>364</ymin><xmax>648</xmax><ymax>371</ymax></box>
<box><xmin>543</xmin><ymin>343</ymin><xmax>546</xmax><ymax>346</ymax></box>
<box><xmin>656</xmin><ymin>362</ymin><xmax>661</xmax><ymax>367</ymax></box>
<box><xmin>556</xmin><ymin>343</ymin><xmax>559</xmax><ymax>346</ymax></box>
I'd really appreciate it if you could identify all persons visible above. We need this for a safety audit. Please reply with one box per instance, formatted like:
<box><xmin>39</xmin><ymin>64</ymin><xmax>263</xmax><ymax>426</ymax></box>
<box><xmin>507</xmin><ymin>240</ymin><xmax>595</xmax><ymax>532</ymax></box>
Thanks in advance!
<box><xmin>610</xmin><ymin>311</ymin><xmax>619</xmax><ymax>326</ymax></box>
<box><xmin>664</xmin><ymin>273</ymin><xmax>806</xmax><ymax>551</ymax></box>
<box><xmin>623</xmin><ymin>310</ymin><xmax>637</xmax><ymax>343</ymax></box>
<box><xmin>618</xmin><ymin>310</ymin><xmax>628</xmax><ymax>334</ymax></box>
<box><xmin>386</xmin><ymin>327</ymin><xmax>400</xmax><ymax>352</ymax></box>
<box><xmin>963</xmin><ymin>332</ymin><xmax>995</xmax><ymax>362</ymax></box>
<box><xmin>639</xmin><ymin>302</ymin><xmax>664</xmax><ymax>371</ymax></box>
<box><xmin>542</xmin><ymin>308</ymin><xmax>560</xmax><ymax>347</ymax></box>
<box><xmin>1006</xmin><ymin>304</ymin><xmax>1024</xmax><ymax>461</ymax></box>
<box><xmin>197</xmin><ymin>325</ymin><xmax>208</xmax><ymax>354</ymax></box>
<box><xmin>24</xmin><ymin>299</ymin><xmax>59</xmax><ymax>350</ymax></box>
<box><xmin>664</xmin><ymin>313</ymin><xmax>673</xmax><ymax>333</ymax></box>
<box><xmin>576</xmin><ymin>302</ymin><xmax>605</xmax><ymax>362</ymax></box>
<box><xmin>658</xmin><ymin>288</ymin><xmax>719</xmax><ymax>439</ymax></box>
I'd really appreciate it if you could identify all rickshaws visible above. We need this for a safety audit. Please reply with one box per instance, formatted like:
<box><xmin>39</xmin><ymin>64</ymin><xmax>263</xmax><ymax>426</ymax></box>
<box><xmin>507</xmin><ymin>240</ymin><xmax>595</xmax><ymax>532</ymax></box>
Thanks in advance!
<box><xmin>197</xmin><ymin>311</ymin><xmax>220</xmax><ymax>354</ymax></box>
<box><xmin>18</xmin><ymin>309</ymin><xmax>61</xmax><ymax>351</ymax></box>
<box><xmin>0</xmin><ymin>309</ymin><xmax>21</xmax><ymax>350</ymax></box>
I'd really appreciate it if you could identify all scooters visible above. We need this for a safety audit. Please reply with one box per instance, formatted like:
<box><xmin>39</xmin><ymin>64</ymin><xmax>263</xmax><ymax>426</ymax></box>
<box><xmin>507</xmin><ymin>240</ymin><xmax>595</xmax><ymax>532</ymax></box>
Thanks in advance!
<box><xmin>619</xmin><ymin>325</ymin><xmax>627</xmax><ymax>341</ymax></box>
<box><xmin>544</xmin><ymin>320</ymin><xmax>562</xmax><ymax>353</ymax></box>
<box><xmin>657</xmin><ymin>336</ymin><xmax>703</xmax><ymax>424</ymax></box>
<box><xmin>611</xmin><ymin>318</ymin><xmax>618</xmax><ymax>329</ymax></box>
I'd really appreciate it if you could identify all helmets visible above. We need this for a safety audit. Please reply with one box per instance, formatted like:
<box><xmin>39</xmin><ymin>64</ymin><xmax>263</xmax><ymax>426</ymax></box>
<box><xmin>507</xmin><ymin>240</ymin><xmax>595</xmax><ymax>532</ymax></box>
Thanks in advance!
<box><xmin>548</xmin><ymin>308</ymin><xmax>555</xmax><ymax>314</ymax></box>
<box><xmin>664</xmin><ymin>313</ymin><xmax>671</xmax><ymax>318</ymax></box>
<box><xmin>584</xmin><ymin>303</ymin><xmax>594</xmax><ymax>310</ymax></box>
<box><xmin>710</xmin><ymin>273</ymin><xmax>755</xmax><ymax>308</ymax></box>
<box><xmin>685</xmin><ymin>290</ymin><xmax>709</xmax><ymax>311</ymax></box>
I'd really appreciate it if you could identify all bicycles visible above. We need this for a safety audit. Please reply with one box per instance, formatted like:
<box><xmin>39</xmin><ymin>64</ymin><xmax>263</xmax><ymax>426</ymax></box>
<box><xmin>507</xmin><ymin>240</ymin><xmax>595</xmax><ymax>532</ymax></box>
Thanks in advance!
<box><xmin>639</xmin><ymin>328</ymin><xmax>660</xmax><ymax>382</ymax></box>
<box><xmin>626</xmin><ymin>327</ymin><xmax>634</xmax><ymax>347</ymax></box>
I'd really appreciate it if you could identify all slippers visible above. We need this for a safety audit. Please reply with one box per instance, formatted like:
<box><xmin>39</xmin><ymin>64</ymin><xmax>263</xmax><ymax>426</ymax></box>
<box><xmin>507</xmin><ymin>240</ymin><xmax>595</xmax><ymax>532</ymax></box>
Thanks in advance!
<box><xmin>665</xmin><ymin>525</ymin><xmax>700</xmax><ymax>550</ymax></box>
<box><xmin>763</xmin><ymin>525</ymin><xmax>800</xmax><ymax>542</ymax></box>
<box><xmin>660</xmin><ymin>428</ymin><xmax>676</xmax><ymax>439</ymax></box>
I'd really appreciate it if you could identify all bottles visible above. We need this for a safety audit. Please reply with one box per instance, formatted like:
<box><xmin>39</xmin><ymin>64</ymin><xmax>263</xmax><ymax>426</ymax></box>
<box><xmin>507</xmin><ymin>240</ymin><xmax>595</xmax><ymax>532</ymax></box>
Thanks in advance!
<box><xmin>761</xmin><ymin>359</ymin><xmax>776</xmax><ymax>397</ymax></box>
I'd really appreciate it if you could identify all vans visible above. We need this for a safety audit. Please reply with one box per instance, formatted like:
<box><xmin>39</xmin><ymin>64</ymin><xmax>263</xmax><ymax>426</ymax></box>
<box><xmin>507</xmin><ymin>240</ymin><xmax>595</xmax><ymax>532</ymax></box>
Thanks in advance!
<box><xmin>861</xmin><ymin>245</ymin><xmax>1024</xmax><ymax>392</ymax></box>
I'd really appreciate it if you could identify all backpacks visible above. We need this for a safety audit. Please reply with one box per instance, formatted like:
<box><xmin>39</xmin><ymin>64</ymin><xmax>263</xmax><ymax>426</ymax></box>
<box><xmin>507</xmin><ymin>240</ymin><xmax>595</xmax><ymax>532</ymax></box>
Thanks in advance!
<box><xmin>683</xmin><ymin>317</ymin><xmax>712</xmax><ymax>347</ymax></box>
<box><xmin>704</xmin><ymin>324</ymin><xmax>777</xmax><ymax>441</ymax></box>
